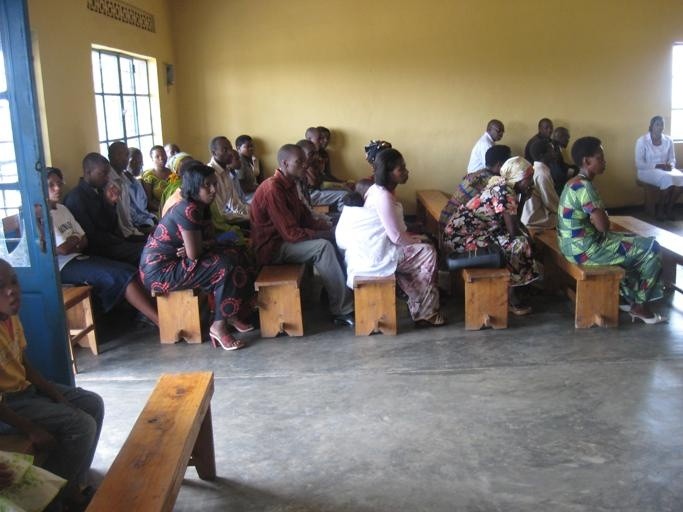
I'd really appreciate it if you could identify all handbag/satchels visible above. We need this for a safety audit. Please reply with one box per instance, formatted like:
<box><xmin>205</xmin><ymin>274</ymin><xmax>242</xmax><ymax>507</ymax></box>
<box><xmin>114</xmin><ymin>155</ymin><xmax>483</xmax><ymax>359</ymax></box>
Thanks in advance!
<box><xmin>447</xmin><ymin>247</ymin><xmax>508</xmax><ymax>271</ymax></box>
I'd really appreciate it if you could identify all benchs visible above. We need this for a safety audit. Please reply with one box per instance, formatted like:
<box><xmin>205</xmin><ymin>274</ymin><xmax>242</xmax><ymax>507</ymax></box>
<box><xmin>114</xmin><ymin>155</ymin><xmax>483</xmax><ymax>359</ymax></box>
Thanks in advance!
<box><xmin>0</xmin><ymin>432</ymin><xmax>40</xmax><ymax>469</ymax></box>
<box><xmin>415</xmin><ymin>189</ymin><xmax>512</xmax><ymax>330</ymax></box>
<box><xmin>254</xmin><ymin>205</ymin><xmax>330</xmax><ymax>338</ymax></box>
<box><xmin>610</xmin><ymin>216</ymin><xmax>683</xmax><ymax>294</ymax></box>
<box><xmin>87</xmin><ymin>371</ymin><xmax>216</xmax><ymax>512</ymax></box>
<box><xmin>156</xmin><ymin>288</ymin><xmax>204</xmax><ymax>342</ymax></box>
<box><xmin>535</xmin><ymin>229</ymin><xmax>626</xmax><ymax>329</ymax></box>
<box><xmin>354</xmin><ymin>276</ymin><xmax>397</xmax><ymax>336</ymax></box>
<box><xmin>637</xmin><ymin>180</ymin><xmax>659</xmax><ymax>217</ymax></box>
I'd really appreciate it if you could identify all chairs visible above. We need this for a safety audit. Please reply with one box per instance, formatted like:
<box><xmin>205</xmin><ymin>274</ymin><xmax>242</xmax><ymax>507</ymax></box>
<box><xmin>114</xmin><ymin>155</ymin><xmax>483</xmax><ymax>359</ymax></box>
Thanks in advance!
<box><xmin>61</xmin><ymin>283</ymin><xmax>97</xmax><ymax>375</ymax></box>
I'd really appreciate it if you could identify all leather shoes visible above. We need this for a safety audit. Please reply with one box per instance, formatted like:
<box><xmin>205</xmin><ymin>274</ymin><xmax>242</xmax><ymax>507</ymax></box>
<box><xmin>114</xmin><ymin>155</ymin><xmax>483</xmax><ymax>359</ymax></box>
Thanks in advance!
<box><xmin>506</xmin><ymin>300</ymin><xmax>535</xmax><ymax>317</ymax></box>
<box><xmin>333</xmin><ymin>315</ymin><xmax>354</xmax><ymax>327</ymax></box>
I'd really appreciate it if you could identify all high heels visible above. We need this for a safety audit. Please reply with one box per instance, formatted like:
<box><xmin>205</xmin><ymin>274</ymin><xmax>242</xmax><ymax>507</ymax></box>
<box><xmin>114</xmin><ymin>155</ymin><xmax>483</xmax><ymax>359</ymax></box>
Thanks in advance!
<box><xmin>630</xmin><ymin>307</ymin><xmax>667</xmax><ymax>324</ymax></box>
<box><xmin>210</xmin><ymin>333</ymin><xmax>245</xmax><ymax>350</ymax></box>
<box><xmin>619</xmin><ymin>304</ymin><xmax>632</xmax><ymax>312</ymax></box>
<box><xmin>415</xmin><ymin>314</ymin><xmax>448</xmax><ymax>328</ymax></box>
<box><xmin>228</xmin><ymin>320</ymin><xmax>255</xmax><ymax>334</ymax></box>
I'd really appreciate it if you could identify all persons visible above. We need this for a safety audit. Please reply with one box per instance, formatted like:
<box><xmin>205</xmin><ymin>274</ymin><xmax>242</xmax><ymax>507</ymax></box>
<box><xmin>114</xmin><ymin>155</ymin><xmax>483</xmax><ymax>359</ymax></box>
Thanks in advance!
<box><xmin>519</xmin><ymin>139</ymin><xmax>560</xmax><ymax>230</ymax></box>
<box><xmin>224</xmin><ymin>151</ymin><xmax>250</xmax><ymax>211</ymax></box>
<box><xmin>0</xmin><ymin>450</ymin><xmax>67</xmax><ymax>512</ymax></box>
<box><xmin>0</xmin><ymin>252</ymin><xmax>100</xmax><ymax>510</ymax></box>
<box><xmin>444</xmin><ymin>156</ymin><xmax>544</xmax><ymax>317</ymax></box>
<box><xmin>347</xmin><ymin>148</ymin><xmax>448</xmax><ymax>329</ymax></box>
<box><xmin>635</xmin><ymin>116</ymin><xmax>683</xmax><ymax>221</ymax></box>
<box><xmin>156</xmin><ymin>153</ymin><xmax>250</xmax><ymax>246</ymax></box>
<box><xmin>523</xmin><ymin>118</ymin><xmax>554</xmax><ymax>193</ymax></box>
<box><xmin>68</xmin><ymin>152</ymin><xmax>157</xmax><ymax>329</ymax></box>
<box><xmin>205</xmin><ymin>135</ymin><xmax>252</xmax><ymax>232</ymax></box>
<box><xmin>142</xmin><ymin>143</ymin><xmax>171</xmax><ymax>214</ymax></box>
<box><xmin>557</xmin><ymin>136</ymin><xmax>670</xmax><ymax>326</ymax></box>
<box><xmin>104</xmin><ymin>142</ymin><xmax>156</xmax><ymax>242</ymax></box>
<box><xmin>546</xmin><ymin>128</ymin><xmax>577</xmax><ymax>200</ymax></box>
<box><xmin>334</xmin><ymin>193</ymin><xmax>364</xmax><ymax>248</ymax></box>
<box><xmin>235</xmin><ymin>135</ymin><xmax>267</xmax><ymax>191</ymax></box>
<box><xmin>161</xmin><ymin>161</ymin><xmax>236</xmax><ymax>249</ymax></box>
<box><xmin>137</xmin><ymin>165</ymin><xmax>255</xmax><ymax>351</ymax></box>
<box><xmin>124</xmin><ymin>148</ymin><xmax>159</xmax><ymax>227</ymax></box>
<box><xmin>166</xmin><ymin>143</ymin><xmax>182</xmax><ymax>170</ymax></box>
<box><xmin>162</xmin><ymin>160</ymin><xmax>254</xmax><ymax>321</ymax></box>
<box><xmin>435</xmin><ymin>145</ymin><xmax>536</xmax><ymax>261</ymax></box>
<box><xmin>45</xmin><ymin>162</ymin><xmax>161</xmax><ymax>329</ymax></box>
<box><xmin>304</xmin><ymin>127</ymin><xmax>353</xmax><ymax>216</ymax></box>
<box><xmin>292</xmin><ymin>142</ymin><xmax>341</xmax><ymax>227</ymax></box>
<box><xmin>249</xmin><ymin>144</ymin><xmax>356</xmax><ymax>328</ymax></box>
<box><xmin>466</xmin><ymin>120</ymin><xmax>504</xmax><ymax>175</ymax></box>
<box><xmin>356</xmin><ymin>140</ymin><xmax>436</xmax><ymax>299</ymax></box>
<box><xmin>316</xmin><ymin>125</ymin><xmax>355</xmax><ymax>192</ymax></box>
